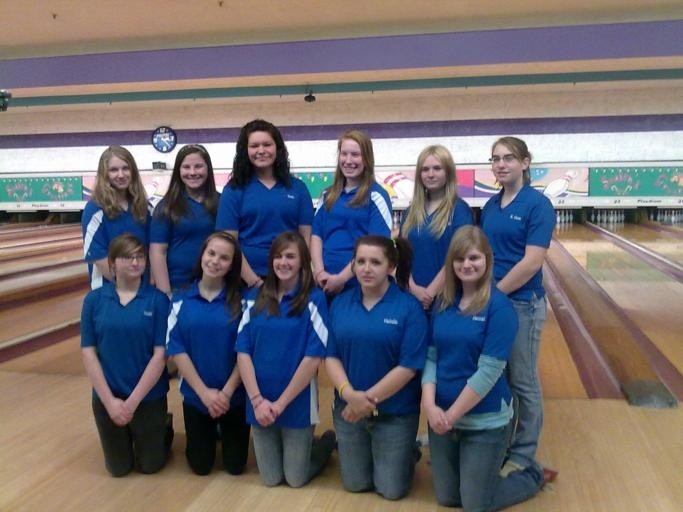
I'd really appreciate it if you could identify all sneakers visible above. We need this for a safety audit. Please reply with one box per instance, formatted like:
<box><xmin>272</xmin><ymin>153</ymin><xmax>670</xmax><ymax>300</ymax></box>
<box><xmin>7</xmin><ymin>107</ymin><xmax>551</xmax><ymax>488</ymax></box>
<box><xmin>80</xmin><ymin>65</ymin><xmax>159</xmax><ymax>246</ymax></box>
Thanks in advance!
<box><xmin>501</xmin><ymin>460</ymin><xmax>525</xmax><ymax>478</ymax></box>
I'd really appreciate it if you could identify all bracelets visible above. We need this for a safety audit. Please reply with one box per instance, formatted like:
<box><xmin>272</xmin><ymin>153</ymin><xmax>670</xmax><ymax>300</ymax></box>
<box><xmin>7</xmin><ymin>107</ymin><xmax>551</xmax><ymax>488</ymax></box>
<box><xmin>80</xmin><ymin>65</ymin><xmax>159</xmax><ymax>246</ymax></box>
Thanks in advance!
<box><xmin>250</xmin><ymin>393</ymin><xmax>264</xmax><ymax>410</ymax></box>
<box><xmin>248</xmin><ymin>276</ymin><xmax>261</xmax><ymax>289</ymax></box>
<box><xmin>338</xmin><ymin>381</ymin><xmax>349</xmax><ymax>399</ymax></box>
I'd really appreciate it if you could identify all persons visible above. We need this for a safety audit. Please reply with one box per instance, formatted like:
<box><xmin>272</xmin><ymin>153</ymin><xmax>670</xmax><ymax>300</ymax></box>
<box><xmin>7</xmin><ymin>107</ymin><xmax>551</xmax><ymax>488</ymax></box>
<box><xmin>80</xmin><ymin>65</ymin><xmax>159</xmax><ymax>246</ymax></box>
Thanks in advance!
<box><xmin>398</xmin><ymin>145</ymin><xmax>475</xmax><ymax>311</ymax></box>
<box><xmin>149</xmin><ymin>144</ymin><xmax>222</xmax><ymax>301</ymax></box>
<box><xmin>324</xmin><ymin>236</ymin><xmax>430</xmax><ymax>501</ymax></box>
<box><xmin>80</xmin><ymin>231</ymin><xmax>174</xmax><ymax>479</ymax></box>
<box><xmin>164</xmin><ymin>231</ymin><xmax>251</xmax><ymax>476</ymax></box>
<box><xmin>232</xmin><ymin>230</ymin><xmax>337</xmax><ymax>489</ymax></box>
<box><xmin>214</xmin><ymin>119</ymin><xmax>314</xmax><ymax>290</ymax></box>
<box><xmin>309</xmin><ymin>129</ymin><xmax>394</xmax><ymax>296</ymax></box>
<box><xmin>480</xmin><ymin>136</ymin><xmax>557</xmax><ymax>478</ymax></box>
<box><xmin>421</xmin><ymin>225</ymin><xmax>559</xmax><ymax>512</ymax></box>
<box><xmin>81</xmin><ymin>145</ymin><xmax>154</xmax><ymax>291</ymax></box>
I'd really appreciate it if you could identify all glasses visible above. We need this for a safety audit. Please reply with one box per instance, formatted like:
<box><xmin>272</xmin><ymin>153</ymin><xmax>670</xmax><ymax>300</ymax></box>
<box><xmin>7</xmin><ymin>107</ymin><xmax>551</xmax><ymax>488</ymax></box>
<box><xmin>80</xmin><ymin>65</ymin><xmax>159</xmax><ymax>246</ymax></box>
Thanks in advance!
<box><xmin>119</xmin><ymin>253</ymin><xmax>146</xmax><ymax>263</ymax></box>
<box><xmin>488</xmin><ymin>154</ymin><xmax>515</xmax><ymax>164</ymax></box>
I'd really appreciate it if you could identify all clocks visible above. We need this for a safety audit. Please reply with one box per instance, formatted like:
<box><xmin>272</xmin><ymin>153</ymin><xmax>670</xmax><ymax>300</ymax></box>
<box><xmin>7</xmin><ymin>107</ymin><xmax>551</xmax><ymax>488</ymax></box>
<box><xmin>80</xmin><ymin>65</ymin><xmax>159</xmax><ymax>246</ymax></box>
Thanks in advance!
<box><xmin>151</xmin><ymin>125</ymin><xmax>177</xmax><ymax>155</ymax></box>
